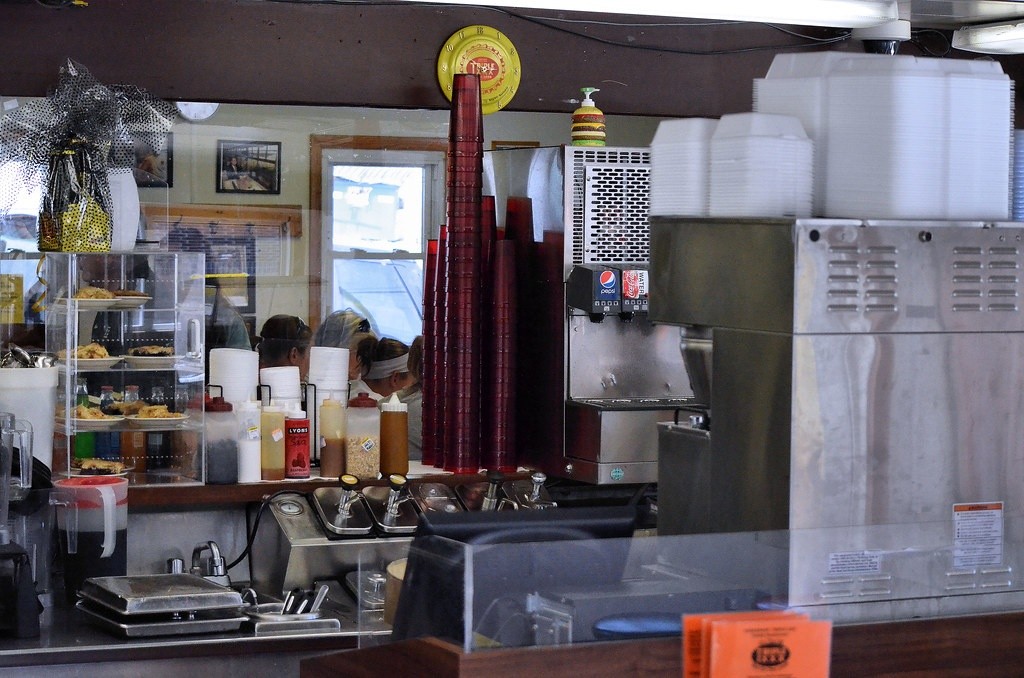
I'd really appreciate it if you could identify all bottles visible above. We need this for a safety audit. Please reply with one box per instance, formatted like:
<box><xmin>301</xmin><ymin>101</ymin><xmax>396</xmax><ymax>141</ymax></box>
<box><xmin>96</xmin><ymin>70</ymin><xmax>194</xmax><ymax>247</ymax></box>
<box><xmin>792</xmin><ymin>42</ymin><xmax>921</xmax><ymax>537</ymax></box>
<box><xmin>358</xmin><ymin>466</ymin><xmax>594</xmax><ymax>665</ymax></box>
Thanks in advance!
<box><xmin>381</xmin><ymin>393</ymin><xmax>409</xmax><ymax>476</ymax></box>
<box><xmin>52</xmin><ymin>377</ymin><xmax>198</xmax><ymax>485</ymax></box>
<box><xmin>319</xmin><ymin>388</ymin><xmax>345</xmax><ymax>477</ymax></box>
<box><xmin>345</xmin><ymin>393</ymin><xmax>380</xmax><ymax>480</ymax></box>
<box><xmin>180</xmin><ymin>391</ymin><xmax>310</xmax><ymax>485</ymax></box>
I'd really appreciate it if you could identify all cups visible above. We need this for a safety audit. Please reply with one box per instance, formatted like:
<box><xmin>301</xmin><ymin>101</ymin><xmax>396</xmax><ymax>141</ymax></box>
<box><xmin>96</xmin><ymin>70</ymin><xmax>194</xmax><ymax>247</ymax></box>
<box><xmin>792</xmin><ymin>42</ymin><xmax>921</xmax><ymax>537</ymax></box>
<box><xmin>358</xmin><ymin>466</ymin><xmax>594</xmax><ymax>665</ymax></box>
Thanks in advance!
<box><xmin>420</xmin><ymin>73</ymin><xmax>549</xmax><ymax>473</ymax></box>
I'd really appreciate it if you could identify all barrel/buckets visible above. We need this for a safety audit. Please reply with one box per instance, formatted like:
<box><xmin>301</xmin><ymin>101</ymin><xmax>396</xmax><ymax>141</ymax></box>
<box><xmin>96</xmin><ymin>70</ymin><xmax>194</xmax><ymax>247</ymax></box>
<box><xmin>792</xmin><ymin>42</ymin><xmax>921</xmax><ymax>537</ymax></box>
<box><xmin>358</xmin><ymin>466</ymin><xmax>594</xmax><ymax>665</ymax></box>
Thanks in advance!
<box><xmin>0</xmin><ymin>367</ymin><xmax>58</xmax><ymax>472</ymax></box>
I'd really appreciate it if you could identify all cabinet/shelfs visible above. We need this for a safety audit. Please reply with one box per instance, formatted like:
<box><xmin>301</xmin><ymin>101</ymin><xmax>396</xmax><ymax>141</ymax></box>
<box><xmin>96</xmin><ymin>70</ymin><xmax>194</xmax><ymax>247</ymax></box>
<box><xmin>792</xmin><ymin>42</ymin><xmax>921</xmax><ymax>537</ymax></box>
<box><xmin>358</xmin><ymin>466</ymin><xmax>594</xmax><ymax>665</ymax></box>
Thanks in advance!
<box><xmin>46</xmin><ymin>248</ymin><xmax>206</xmax><ymax>488</ymax></box>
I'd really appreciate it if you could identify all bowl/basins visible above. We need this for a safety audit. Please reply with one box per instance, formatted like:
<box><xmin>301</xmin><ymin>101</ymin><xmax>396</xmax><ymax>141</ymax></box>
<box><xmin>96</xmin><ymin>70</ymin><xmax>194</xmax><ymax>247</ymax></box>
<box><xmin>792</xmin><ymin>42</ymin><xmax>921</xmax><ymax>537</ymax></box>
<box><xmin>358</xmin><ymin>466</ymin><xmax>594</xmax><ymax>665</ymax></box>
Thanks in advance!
<box><xmin>648</xmin><ymin>51</ymin><xmax>1017</xmax><ymax>222</ymax></box>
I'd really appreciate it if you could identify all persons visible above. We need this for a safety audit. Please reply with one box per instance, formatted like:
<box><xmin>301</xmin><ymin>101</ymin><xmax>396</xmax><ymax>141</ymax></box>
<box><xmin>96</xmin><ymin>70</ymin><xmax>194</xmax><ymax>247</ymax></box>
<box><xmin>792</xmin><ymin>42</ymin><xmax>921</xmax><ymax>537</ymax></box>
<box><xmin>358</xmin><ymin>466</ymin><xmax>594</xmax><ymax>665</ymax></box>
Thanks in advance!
<box><xmin>377</xmin><ymin>336</ymin><xmax>423</xmax><ymax>461</ymax></box>
<box><xmin>257</xmin><ymin>314</ymin><xmax>313</xmax><ymax>381</ymax></box>
<box><xmin>349</xmin><ymin>337</ymin><xmax>415</xmax><ymax>401</ymax></box>
<box><xmin>301</xmin><ymin>310</ymin><xmax>378</xmax><ymax>399</ymax></box>
<box><xmin>77</xmin><ymin>254</ymin><xmax>165</xmax><ymax>398</ymax></box>
<box><xmin>123</xmin><ymin>228</ymin><xmax>252</xmax><ymax>413</ymax></box>
<box><xmin>227</xmin><ymin>157</ymin><xmax>240</xmax><ymax>179</ymax></box>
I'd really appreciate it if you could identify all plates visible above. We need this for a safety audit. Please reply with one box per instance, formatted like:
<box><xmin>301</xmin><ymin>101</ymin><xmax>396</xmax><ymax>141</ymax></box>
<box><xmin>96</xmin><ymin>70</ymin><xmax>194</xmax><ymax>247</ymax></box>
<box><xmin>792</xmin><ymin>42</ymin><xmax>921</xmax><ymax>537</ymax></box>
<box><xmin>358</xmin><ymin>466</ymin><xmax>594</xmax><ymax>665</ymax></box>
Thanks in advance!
<box><xmin>119</xmin><ymin>354</ymin><xmax>185</xmax><ymax>367</ymax></box>
<box><xmin>115</xmin><ymin>296</ymin><xmax>153</xmax><ymax>308</ymax></box>
<box><xmin>53</xmin><ymin>296</ymin><xmax>122</xmax><ymax>308</ymax></box>
<box><xmin>58</xmin><ymin>356</ymin><xmax>125</xmax><ymax>369</ymax></box>
<box><xmin>208</xmin><ymin>347</ymin><xmax>349</xmax><ymax>460</ymax></box>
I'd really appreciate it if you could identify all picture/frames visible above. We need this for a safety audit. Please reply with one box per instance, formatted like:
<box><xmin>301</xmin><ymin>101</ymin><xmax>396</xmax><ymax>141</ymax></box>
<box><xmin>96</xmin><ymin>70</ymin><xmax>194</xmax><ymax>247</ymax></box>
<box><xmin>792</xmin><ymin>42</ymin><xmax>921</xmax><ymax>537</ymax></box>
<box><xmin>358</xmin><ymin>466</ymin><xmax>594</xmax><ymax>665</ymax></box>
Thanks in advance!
<box><xmin>119</xmin><ymin>131</ymin><xmax>174</xmax><ymax>187</ymax></box>
<box><xmin>216</xmin><ymin>140</ymin><xmax>282</xmax><ymax>194</ymax></box>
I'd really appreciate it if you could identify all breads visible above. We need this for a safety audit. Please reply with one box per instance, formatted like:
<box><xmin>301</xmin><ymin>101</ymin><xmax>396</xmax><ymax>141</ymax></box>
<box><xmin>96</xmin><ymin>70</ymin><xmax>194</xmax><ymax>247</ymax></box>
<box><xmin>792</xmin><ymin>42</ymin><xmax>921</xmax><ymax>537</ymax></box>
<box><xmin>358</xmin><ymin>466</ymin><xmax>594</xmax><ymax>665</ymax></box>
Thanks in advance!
<box><xmin>57</xmin><ymin>286</ymin><xmax>174</xmax><ymax>473</ymax></box>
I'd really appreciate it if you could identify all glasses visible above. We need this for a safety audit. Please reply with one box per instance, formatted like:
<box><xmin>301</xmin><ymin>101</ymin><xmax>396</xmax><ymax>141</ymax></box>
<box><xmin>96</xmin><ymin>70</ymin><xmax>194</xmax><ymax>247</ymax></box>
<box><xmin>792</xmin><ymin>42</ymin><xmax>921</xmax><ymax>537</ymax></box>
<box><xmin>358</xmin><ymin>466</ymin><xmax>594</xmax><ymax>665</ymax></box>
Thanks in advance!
<box><xmin>353</xmin><ymin>319</ymin><xmax>370</xmax><ymax>335</ymax></box>
<box><xmin>291</xmin><ymin>316</ymin><xmax>305</xmax><ymax>337</ymax></box>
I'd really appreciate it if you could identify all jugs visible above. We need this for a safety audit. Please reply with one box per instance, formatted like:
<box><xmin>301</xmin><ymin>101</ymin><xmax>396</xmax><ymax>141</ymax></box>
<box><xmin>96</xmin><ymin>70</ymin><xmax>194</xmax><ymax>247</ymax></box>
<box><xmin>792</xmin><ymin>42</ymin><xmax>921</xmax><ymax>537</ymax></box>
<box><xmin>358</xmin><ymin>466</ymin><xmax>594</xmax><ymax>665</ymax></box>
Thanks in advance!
<box><xmin>52</xmin><ymin>477</ymin><xmax>129</xmax><ymax>608</ymax></box>
<box><xmin>0</xmin><ymin>412</ymin><xmax>34</xmax><ymax>546</ymax></box>
